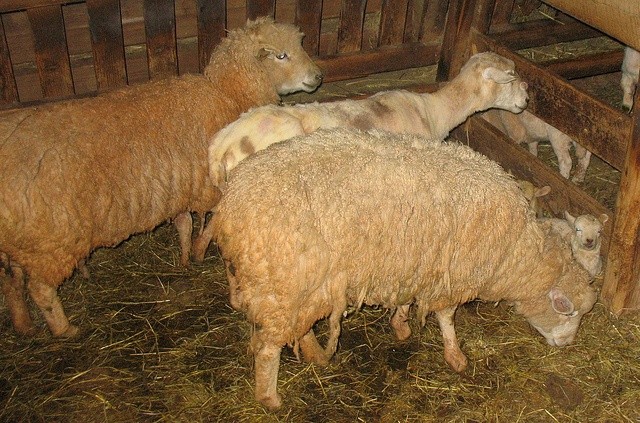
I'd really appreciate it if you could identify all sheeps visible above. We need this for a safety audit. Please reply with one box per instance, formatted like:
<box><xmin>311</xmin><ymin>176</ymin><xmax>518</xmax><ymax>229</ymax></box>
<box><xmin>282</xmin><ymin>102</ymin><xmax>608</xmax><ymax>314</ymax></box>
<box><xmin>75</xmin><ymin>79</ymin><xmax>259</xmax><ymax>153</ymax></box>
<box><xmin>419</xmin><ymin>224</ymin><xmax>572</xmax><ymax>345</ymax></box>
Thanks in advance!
<box><xmin>193</xmin><ymin>125</ymin><xmax>598</xmax><ymax>413</ymax></box>
<box><xmin>536</xmin><ymin>208</ymin><xmax>610</xmax><ymax>280</ymax></box>
<box><xmin>209</xmin><ymin>49</ymin><xmax>531</xmax><ymax>197</ymax></box>
<box><xmin>478</xmin><ymin>106</ymin><xmax>594</xmax><ymax>188</ymax></box>
<box><xmin>618</xmin><ymin>42</ymin><xmax>640</xmax><ymax>116</ymax></box>
<box><xmin>1</xmin><ymin>9</ymin><xmax>328</xmax><ymax>342</ymax></box>
